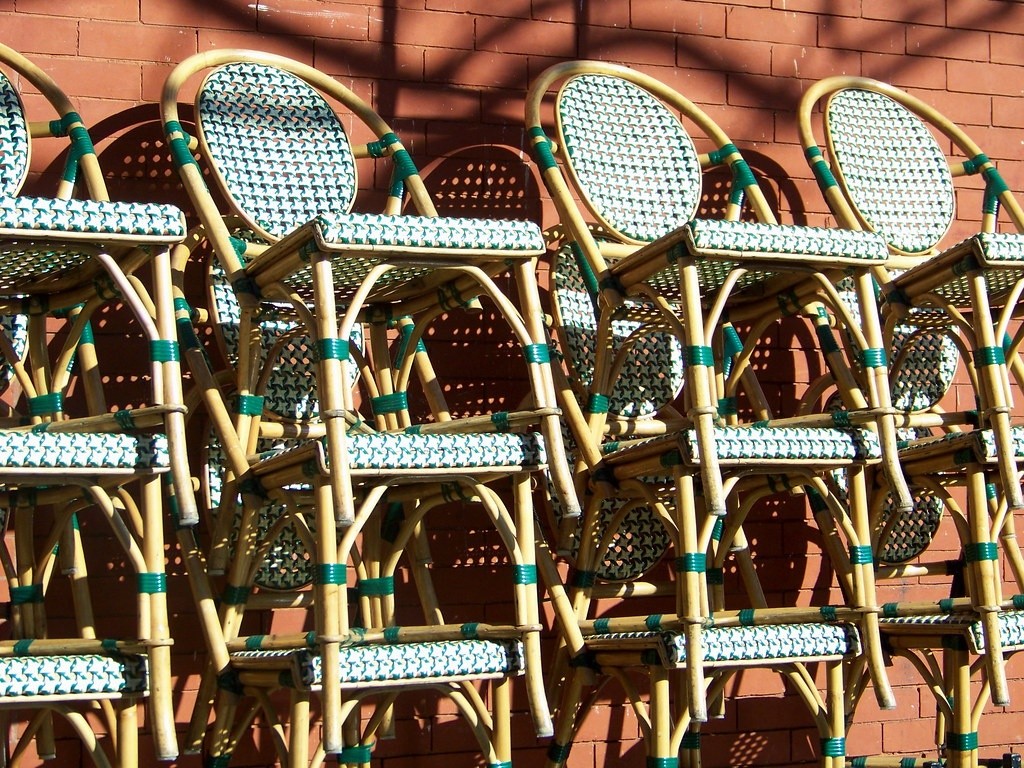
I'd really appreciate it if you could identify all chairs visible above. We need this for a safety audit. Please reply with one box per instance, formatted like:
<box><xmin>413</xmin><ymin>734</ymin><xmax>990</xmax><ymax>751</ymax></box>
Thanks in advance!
<box><xmin>0</xmin><ymin>39</ymin><xmax>1024</xmax><ymax>768</ymax></box>
